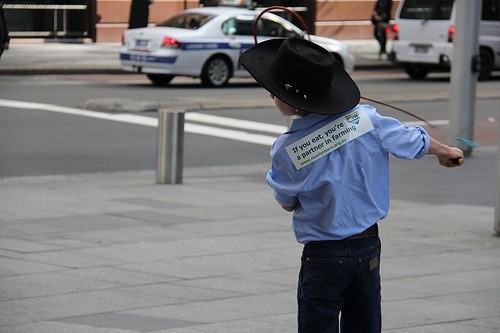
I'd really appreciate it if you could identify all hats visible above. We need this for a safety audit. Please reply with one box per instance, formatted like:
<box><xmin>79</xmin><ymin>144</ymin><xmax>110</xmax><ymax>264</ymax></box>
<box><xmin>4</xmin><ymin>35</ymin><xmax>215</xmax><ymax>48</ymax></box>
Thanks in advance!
<box><xmin>238</xmin><ymin>37</ymin><xmax>361</xmax><ymax>114</ymax></box>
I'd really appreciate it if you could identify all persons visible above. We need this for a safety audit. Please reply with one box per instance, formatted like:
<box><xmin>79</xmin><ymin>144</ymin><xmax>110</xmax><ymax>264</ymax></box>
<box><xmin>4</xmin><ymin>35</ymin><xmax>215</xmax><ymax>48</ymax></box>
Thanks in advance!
<box><xmin>370</xmin><ymin>0</ymin><xmax>393</xmax><ymax>60</ymax></box>
<box><xmin>238</xmin><ymin>36</ymin><xmax>464</xmax><ymax>333</ymax></box>
<box><xmin>0</xmin><ymin>0</ymin><xmax>10</xmax><ymax>57</ymax></box>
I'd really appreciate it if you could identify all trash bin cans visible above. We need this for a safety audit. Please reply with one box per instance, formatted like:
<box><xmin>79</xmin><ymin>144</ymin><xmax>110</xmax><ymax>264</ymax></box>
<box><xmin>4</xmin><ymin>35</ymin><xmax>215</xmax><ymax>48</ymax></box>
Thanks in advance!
<box><xmin>155</xmin><ymin>108</ymin><xmax>185</xmax><ymax>185</ymax></box>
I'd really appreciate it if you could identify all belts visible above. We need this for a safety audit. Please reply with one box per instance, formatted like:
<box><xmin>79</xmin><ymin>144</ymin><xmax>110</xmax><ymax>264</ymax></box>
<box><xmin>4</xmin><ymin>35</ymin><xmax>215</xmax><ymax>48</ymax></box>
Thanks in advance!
<box><xmin>348</xmin><ymin>227</ymin><xmax>377</xmax><ymax>239</ymax></box>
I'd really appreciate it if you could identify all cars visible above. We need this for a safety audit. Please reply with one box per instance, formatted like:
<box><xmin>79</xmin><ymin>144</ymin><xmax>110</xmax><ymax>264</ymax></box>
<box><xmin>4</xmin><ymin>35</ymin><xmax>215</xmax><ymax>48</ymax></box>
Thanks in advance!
<box><xmin>119</xmin><ymin>7</ymin><xmax>355</xmax><ymax>89</ymax></box>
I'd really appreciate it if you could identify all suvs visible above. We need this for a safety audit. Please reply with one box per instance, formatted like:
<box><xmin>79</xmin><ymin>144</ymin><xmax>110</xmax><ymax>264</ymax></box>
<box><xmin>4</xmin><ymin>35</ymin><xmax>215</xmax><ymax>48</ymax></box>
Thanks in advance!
<box><xmin>385</xmin><ymin>0</ymin><xmax>500</xmax><ymax>80</ymax></box>
